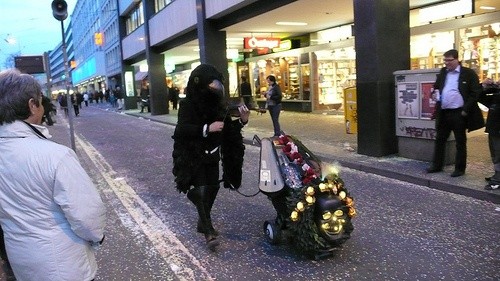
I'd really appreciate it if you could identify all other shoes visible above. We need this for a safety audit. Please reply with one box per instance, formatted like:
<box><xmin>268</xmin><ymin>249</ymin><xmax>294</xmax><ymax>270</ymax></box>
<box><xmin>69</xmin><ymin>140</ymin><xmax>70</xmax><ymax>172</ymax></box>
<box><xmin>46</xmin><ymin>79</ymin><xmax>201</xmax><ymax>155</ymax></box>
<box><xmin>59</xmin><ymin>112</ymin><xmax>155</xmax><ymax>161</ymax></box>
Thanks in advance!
<box><xmin>450</xmin><ymin>170</ymin><xmax>465</xmax><ymax>177</ymax></box>
<box><xmin>484</xmin><ymin>175</ymin><xmax>500</xmax><ymax>181</ymax></box>
<box><xmin>426</xmin><ymin>166</ymin><xmax>444</xmax><ymax>173</ymax></box>
<box><xmin>207</xmin><ymin>235</ymin><xmax>219</xmax><ymax>251</ymax></box>
<box><xmin>484</xmin><ymin>182</ymin><xmax>500</xmax><ymax>190</ymax></box>
<box><xmin>198</xmin><ymin>225</ymin><xmax>218</xmax><ymax>235</ymax></box>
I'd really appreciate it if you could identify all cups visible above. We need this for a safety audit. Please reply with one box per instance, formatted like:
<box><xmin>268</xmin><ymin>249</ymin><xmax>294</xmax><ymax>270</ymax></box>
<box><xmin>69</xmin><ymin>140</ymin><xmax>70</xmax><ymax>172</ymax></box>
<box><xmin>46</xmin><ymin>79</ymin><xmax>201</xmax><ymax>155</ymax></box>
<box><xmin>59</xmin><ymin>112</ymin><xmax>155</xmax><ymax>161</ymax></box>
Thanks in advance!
<box><xmin>434</xmin><ymin>89</ymin><xmax>440</xmax><ymax>101</ymax></box>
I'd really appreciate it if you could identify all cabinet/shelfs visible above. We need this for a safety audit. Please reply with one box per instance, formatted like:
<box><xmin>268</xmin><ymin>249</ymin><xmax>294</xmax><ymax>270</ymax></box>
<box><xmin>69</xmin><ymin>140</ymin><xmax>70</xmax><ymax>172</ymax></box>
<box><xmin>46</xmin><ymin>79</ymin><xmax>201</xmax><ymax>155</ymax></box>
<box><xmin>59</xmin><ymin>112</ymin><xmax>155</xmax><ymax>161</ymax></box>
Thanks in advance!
<box><xmin>287</xmin><ymin>59</ymin><xmax>356</xmax><ymax>94</ymax></box>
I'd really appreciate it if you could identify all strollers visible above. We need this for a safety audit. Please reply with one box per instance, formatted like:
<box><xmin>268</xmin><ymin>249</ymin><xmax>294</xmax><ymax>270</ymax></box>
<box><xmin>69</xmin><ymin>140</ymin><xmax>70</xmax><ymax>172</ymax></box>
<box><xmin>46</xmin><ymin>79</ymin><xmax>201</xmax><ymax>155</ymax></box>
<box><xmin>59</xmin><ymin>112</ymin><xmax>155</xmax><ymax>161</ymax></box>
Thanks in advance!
<box><xmin>251</xmin><ymin>130</ymin><xmax>356</xmax><ymax>258</ymax></box>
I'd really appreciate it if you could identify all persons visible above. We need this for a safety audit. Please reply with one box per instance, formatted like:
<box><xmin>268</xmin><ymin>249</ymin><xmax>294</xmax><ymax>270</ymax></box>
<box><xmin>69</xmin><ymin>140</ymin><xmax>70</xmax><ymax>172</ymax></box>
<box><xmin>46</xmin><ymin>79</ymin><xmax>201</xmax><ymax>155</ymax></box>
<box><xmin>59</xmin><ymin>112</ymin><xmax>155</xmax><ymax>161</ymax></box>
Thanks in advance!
<box><xmin>172</xmin><ymin>64</ymin><xmax>250</xmax><ymax>248</ymax></box>
<box><xmin>94</xmin><ymin>85</ymin><xmax>125</xmax><ymax>110</ymax></box>
<box><xmin>427</xmin><ymin>50</ymin><xmax>485</xmax><ymax>177</ymax></box>
<box><xmin>477</xmin><ymin>83</ymin><xmax>500</xmax><ymax>190</ymax></box>
<box><xmin>241</xmin><ymin>75</ymin><xmax>260</xmax><ymax>110</ymax></box>
<box><xmin>167</xmin><ymin>83</ymin><xmax>180</xmax><ymax>110</ymax></box>
<box><xmin>56</xmin><ymin>90</ymin><xmax>94</xmax><ymax>116</ymax></box>
<box><xmin>261</xmin><ymin>75</ymin><xmax>284</xmax><ymax>136</ymax></box>
<box><xmin>40</xmin><ymin>92</ymin><xmax>55</xmax><ymax>126</ymax></box>
<box><xmin>139</xmin><ymin>81</ymin><xmax>150</xmax><ymax>113</ymax></box>
<box><xmin>0</xmin><ymin>68</ymin><xmax>107</xmax><ymax>281</ymax></box>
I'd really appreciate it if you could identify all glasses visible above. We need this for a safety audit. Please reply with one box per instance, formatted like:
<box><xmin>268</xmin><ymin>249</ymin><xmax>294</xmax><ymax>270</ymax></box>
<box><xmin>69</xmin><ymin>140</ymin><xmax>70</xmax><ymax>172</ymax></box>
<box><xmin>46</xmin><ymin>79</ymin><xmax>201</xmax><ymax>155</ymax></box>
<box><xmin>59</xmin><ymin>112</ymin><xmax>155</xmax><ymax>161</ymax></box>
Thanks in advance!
<box><xmin>444</xmin><ymin>57</ymin><xmax>456</xmax><ymax>61</ymax></box>
